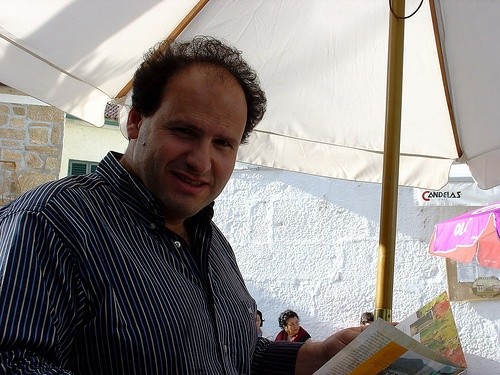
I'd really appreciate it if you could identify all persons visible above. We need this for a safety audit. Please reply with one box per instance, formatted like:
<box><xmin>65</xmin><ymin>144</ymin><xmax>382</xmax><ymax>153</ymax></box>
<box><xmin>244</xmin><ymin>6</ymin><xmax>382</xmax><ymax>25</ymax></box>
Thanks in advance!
<box><xmin>360</xmin><ymin>312</ymin><xmax>375</xmax><ymax>326</ymax></box>
<box><xmin>276</xmin><ymin>309</ymin><xmax>312</xmax><ymax>344</ymax></box>
<box><xmin>256</xmin><ymin>309</ymin><xmax>267</xmax><ymax>338</ymax></box>
<box><xmin>2</xmin><ymin>35</ymin><xmax>401</xmax><ymax>375</ymax></box>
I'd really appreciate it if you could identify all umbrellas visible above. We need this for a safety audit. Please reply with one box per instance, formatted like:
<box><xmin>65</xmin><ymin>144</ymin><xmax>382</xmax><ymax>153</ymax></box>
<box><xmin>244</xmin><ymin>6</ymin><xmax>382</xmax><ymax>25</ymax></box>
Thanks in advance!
<box><xmin>428</xmin><ymin>203</ymin><xmax>500</xmax><ymax>270</ymax></box>
<box><xmin>2</xmin><ymin>0</ymin><xmax>499</xmax><ymax>324</ymax></box>
